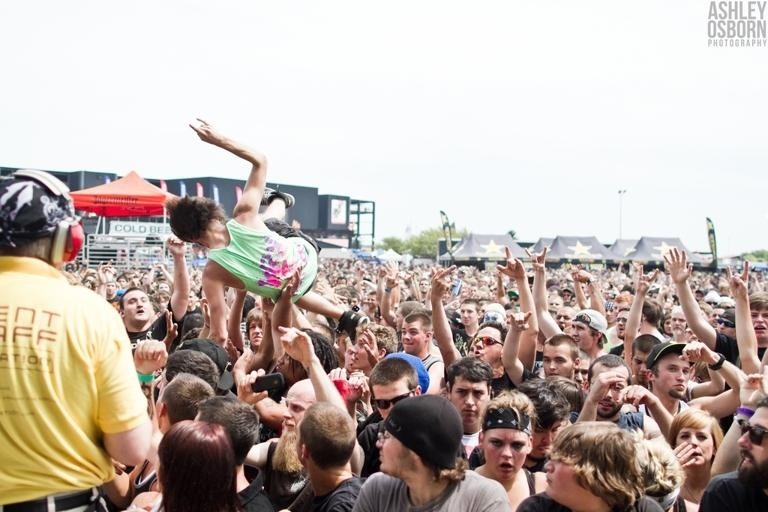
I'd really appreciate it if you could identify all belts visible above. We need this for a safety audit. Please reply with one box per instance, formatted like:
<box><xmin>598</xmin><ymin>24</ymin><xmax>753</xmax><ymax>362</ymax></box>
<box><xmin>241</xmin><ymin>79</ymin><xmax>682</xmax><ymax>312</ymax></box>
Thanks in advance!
<box><xmin>2</xmin><ymin>488</ymin><xmax>104</xmax><ymax>512</ymax></box>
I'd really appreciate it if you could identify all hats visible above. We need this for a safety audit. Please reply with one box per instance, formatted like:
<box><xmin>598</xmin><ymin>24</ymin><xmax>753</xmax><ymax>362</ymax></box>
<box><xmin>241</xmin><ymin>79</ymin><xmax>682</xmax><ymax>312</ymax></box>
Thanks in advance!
<box><xmin>175</xmin><ymin>337</ymin><xmax>234</xmax><ymax>391</ymax></box>
<box><xmin>0</xmin><ymin>170</ymin><xmax>83</xmax><ymax>238</ymax></box>
<box><xmin>645</xmin><ymin>341</ymin><xmax>698</xmax><ymax>370</ymax></box>
<box><xmin>570</xmin><ymin>309</ymin><xmax>608</xmax><ymax>344</ymax></box>
<box><xmin>718</xmin><ymin>307</ymin><xmax>736</xmax><ymax>323</ymax></box>
<box><xmin>383</xmin><ymin>395</ymin><xmax>464</xmax><ymax>470</ymax></box>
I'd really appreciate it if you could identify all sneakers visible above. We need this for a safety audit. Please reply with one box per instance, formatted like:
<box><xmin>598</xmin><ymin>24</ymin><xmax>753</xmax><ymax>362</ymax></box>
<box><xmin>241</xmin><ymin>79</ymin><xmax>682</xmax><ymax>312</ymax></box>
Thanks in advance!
<box><xmin>334</xmin><ymin>305</ymin><xmax>371</xmax><ymax>345</ymax></box>
<box><xmin>258</xmin><ymin>187</ymin><xmax>295</xmax><ymax>210</ymax></box>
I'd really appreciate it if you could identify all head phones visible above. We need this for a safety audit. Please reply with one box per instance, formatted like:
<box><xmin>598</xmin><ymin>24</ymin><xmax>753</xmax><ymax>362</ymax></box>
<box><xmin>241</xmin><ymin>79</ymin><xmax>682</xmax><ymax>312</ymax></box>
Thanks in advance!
<box><xmin>14</xmin><ymin>169</ymin><xmax>84</xmax><ymax>264</ymax></box>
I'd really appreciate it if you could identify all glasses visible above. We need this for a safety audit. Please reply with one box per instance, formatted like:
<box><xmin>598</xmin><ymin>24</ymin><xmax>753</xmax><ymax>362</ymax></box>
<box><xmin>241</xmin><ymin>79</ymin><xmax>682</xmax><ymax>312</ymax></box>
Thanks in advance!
<box><xmin>736</xmin><ymin>416</ymin><xmax>768</xmax><ymax>446</ymax></box>
<box><xmin>372</xmin><ymin>387</ymin><xmax>417</xmax><ymax>410</ymax></box>
<box><xmin>472</xmin><ymin>335</ymin><xmax>505</xmax><ymax>346</ymax></box>
<box><xmin>714</xmin><ymin>317</ymin><xmax>736</xmax><ymax>329</ymax></box>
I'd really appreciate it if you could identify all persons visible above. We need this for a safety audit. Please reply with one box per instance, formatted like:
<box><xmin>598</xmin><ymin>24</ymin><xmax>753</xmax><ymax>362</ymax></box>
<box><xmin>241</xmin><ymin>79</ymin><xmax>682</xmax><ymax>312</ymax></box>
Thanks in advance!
<box><xmin>162</xmin><ymin>115</ymin><xmax>371</xmax><ymax>349</ymax></box>
<box><xmin>1</xmin><ymin>167</ymin><xmax>155</xmax><ymax>510</ymax></box>
<box><xmin>64</xmin><ymin>245</ymin><xmax>767</xmax><ymax>511</ymax></box>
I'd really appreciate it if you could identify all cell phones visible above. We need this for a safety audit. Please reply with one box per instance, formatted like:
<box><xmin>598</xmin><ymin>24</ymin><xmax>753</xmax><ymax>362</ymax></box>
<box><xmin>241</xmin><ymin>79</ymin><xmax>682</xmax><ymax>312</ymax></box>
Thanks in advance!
<box><xmin>250</xmin><ymin>373</ymin><xmax>285</xmax><ymax>392</ymax></box>
